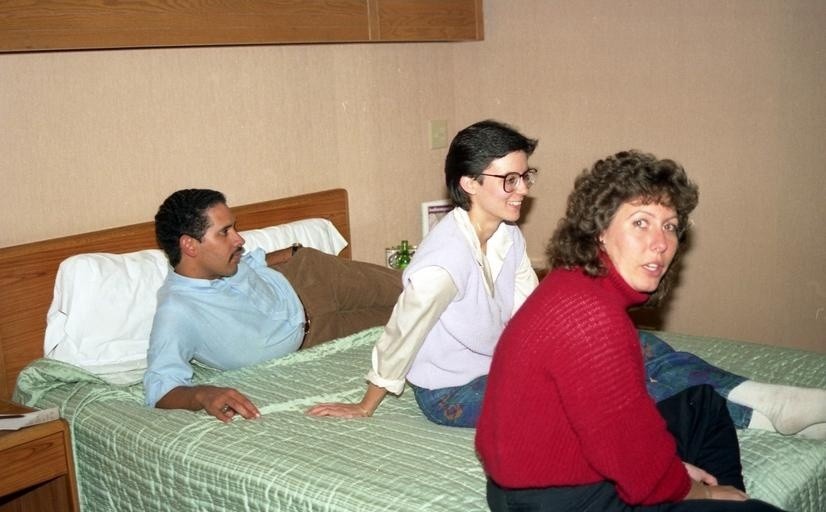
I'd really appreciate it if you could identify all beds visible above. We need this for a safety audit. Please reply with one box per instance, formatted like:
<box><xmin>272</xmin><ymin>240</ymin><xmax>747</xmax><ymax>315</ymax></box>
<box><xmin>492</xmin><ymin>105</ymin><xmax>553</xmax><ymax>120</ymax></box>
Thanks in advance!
<box><xmin>0</xmin><ymin>188</ymin><xmax>826</xmax><ymax>512</ymax></box>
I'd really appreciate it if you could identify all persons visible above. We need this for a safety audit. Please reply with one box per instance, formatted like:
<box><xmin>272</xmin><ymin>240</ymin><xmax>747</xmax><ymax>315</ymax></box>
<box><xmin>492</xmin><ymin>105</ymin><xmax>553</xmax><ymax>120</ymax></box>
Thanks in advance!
<box><xmin>305</xmin><ymin>118</ymin><xmax>826</xmax><ymax>430</ymax></box>
<box><xmin>140</xmin><ymin>188</ymin><xmax>403</xmax><ymax>426</ymax></box>
<box><xmin>472</xmin><ymin>147</ymin><xmax>789</xmax><ymax>511</ymax></box>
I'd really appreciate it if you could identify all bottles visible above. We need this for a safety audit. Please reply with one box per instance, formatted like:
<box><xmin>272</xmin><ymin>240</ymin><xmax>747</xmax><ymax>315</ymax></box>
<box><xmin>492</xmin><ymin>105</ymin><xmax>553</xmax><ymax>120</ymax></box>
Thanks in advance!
<box><xmin>398</xmin><ymin>241</ymin><xmax>411</xmax><ymax>270</ymax></box>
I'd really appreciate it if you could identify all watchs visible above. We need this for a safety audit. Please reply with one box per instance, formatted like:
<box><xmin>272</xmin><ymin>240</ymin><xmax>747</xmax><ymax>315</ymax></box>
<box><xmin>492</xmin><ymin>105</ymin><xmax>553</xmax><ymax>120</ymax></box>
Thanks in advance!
<box><xmin>292</xmin><ymin>242</ymin><xmax>302</xmax><ymax>256</ymax></box>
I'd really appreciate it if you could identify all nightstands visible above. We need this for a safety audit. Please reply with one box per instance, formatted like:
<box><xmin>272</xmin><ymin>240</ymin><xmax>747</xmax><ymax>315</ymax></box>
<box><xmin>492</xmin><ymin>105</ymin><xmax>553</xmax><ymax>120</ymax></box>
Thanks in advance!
<box><xmin>0</xmin><ymin>399</ymin><xmax>82</xmax><ymax>512</ymax></box>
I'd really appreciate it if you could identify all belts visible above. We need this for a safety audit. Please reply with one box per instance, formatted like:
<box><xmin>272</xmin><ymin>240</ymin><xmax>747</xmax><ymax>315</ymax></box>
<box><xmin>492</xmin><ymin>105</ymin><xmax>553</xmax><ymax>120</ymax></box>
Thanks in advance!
<box><xmin>298</xmin><ymin>294</ymin><xmax>310</xmax><ymax>351</ymax></box>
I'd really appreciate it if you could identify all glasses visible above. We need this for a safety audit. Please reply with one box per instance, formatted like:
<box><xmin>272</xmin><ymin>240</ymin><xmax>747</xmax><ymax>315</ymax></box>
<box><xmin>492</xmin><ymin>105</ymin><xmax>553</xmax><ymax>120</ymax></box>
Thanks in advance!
<box><xmin>480</xmin><ymin>168</ymin><xmax>537</xmax><ymax>193</ymax></box>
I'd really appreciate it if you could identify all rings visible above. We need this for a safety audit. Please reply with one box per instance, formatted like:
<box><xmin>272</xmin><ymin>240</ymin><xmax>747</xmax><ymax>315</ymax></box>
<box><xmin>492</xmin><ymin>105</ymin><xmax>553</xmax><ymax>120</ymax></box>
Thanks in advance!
<box><xmin>221</xmin><ymin>404</ymin><xmax>229</xmax><ymax>413</ymax></box>
